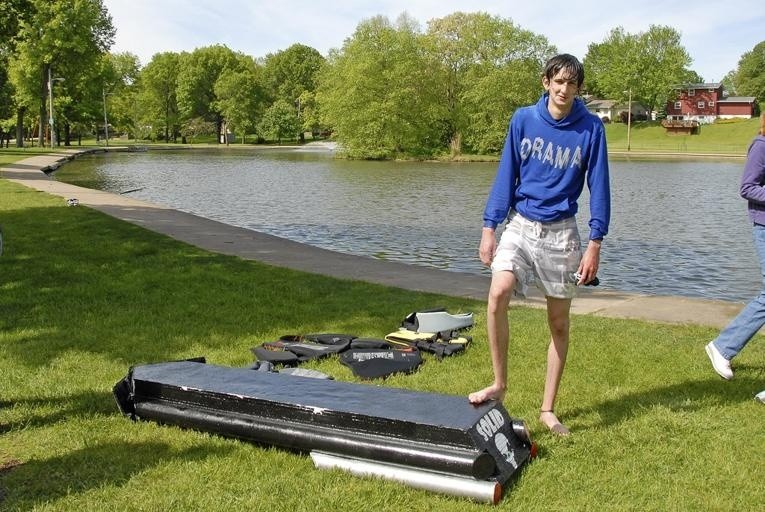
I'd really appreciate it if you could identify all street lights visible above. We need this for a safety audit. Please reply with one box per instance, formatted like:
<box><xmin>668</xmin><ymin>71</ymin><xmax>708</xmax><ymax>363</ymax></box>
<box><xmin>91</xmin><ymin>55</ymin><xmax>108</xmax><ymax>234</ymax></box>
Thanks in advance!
<box><xmin>103</xmin><ymin>92</ymin><xmax>114</xmax><ymax>145</ymax></box>
<box><xmin>622</xmin><ymin>88</ymin><xmax>632</xmax><ymax>151</ymax></box>
<box><xmin>47</xmin><ymin>77</ymin><xmax>65</xmax><ymax>149</ymax></box>
<box><xmin>294</xmin><ymin>97</ymin><xmax>301</xmax><ymax>141</ymax></box>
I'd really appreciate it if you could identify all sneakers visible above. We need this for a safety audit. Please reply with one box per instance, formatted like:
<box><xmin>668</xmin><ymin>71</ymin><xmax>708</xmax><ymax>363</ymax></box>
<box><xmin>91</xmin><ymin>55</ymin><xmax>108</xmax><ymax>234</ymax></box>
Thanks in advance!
<box><xmin>754</xmin><ymin>389</ymin><xmax>765</xmax><ymax>405</ymax></box>
<box><xmin>704</xmin><ymin>340</ymin><xmax>734</xmax><ymax>381</ymax></box>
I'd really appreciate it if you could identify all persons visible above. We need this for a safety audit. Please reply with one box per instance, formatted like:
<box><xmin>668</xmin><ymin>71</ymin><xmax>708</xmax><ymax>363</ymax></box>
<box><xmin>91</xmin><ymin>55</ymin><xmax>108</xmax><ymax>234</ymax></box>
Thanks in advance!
<box><xmin>704</xmin><ymin>112</ymin><xmax>765</xmax><ymax>406</ymax></box>
<box><xmin>467</xmin><ymin>53</ymin><xmax>614</xmax><ymax>440</ymax></box>
